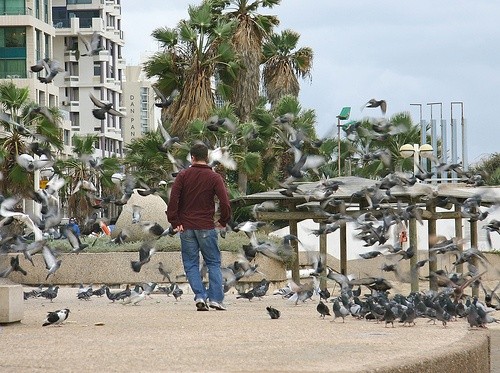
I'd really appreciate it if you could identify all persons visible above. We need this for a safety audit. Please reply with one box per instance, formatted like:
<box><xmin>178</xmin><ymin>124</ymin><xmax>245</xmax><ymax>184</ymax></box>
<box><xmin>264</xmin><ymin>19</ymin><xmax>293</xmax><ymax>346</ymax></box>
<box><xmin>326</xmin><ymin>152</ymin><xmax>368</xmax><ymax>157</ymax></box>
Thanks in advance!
<box><xmin>61</xmin><ymin>210</ymin><xmax>115</xmax><ymax>241</ymax></box>
<box><xmin>166</xmin><ymin>143</ymin><xmax>231</xmax><ymax>311</ymax></box>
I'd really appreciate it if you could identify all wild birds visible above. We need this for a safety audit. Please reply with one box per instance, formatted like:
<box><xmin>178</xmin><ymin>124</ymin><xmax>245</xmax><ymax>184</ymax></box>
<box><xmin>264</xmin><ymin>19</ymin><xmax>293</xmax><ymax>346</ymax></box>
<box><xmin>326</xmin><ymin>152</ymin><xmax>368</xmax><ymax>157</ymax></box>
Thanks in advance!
<box><xmin>41</xmin><ymin>244</ymin><xmax>63</xmax><ymax>280</ymax></box>
<box><xmin>63</xmin><ymin>226</ymin><xmax>89</xmax><ymax>256</ymax></box>
<box><xmin>157</xmin><ymin>262</ymin><xmax>183</xmax><ymax>302</ymax></box>
<box><xmin>0</xmin><ymin>114</ymin><xmax>218</xmax><ymax>267</ymax></box>
<box><xmin>76</xmin><ymin>278</ymin><xmax>158</xmax><ymax>306</ymax></box>
<box><xmin>153</xmin><ymin>86</ymin><xmax>173</xmax><ymax>108</ymax></box>
<box><xmin>77</xmin><ymin>30</ymin><xmax>103</xmax><ymax>56</ymax></box>
<box><xmin>88</xmin><ymin>91</ymin><xmax>126</xmax><ymax>121</ymax></box>
<box><xmin>266</xmin><ymin>305</ymin><xmax>280</xmax><ymax>319</ymax></box>
<box><xmin>30</xmin><ymin>57</ymin><xmax>64</xmax><ymax>83</ymax></box>
<box><xmin>42</xmin><ymin>309</ymin><xmax>71</xmax><ymax>328</ymax></box>
<box><xmin>131</xmin><ymin>205</ymin><xmax>142</xmax><ymax>224</ymax></box>
<box><xmin>24</xmin><ymin>282</ymin><xmax>59</xmax><ymax>303</ymax></box>
<box><xmin>0</xmin><ymin>254</ymin><xmax>27</xmax><ymax>278</ymax></box>
<box><xmin>219</xmin><ymin>99</ymin><xmax>500</xmax><ymax>331</ymax></box>
<box><xmin>143</xmin><ymin>220</ymin><xmax>171</xmax><ymax>240</ymax></box>
<box><xmin>130</xmin><ymin>245</ymin><xmax>156</xmax><ymax>273</ymax></box>
<box><xmin>110</xmin><ymin>231</ymin><xmax>129</xmax><ymax>246</ymax></box>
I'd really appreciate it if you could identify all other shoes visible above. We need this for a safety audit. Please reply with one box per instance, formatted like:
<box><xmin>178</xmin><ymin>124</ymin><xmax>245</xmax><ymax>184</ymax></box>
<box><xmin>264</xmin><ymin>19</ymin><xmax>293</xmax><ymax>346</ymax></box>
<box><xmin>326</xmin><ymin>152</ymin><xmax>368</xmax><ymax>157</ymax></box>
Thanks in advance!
<box><xmin>209</xmin><ymin>301</ymin><xmax>226</xmax><ymax>310</ymax></box>
<box><xmin>195</xmin><ymin>297</ymin><xmax>209</xmax><ymax>311</ymax></box>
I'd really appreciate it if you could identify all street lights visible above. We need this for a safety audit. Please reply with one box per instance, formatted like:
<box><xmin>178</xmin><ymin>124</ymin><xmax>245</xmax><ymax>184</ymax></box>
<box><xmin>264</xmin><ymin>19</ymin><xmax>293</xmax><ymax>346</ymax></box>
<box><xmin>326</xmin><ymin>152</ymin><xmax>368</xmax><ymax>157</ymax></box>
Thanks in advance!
<box><xmin>336</xmin><ymin>104</ymin><xmax>351</xmax><ymax>176</ymax></box>
